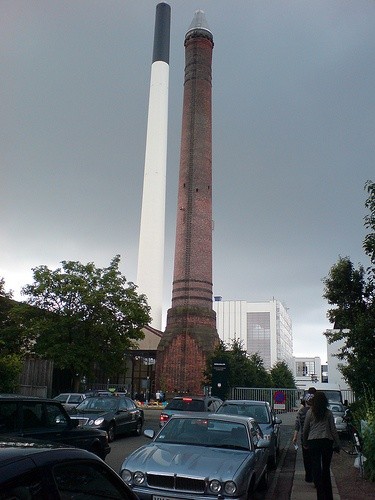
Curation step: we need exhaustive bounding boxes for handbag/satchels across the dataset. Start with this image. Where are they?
[333,441,339,452]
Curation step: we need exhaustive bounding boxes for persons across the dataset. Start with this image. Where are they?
[116,385,128,393]
[292,386,341,500]
[343,400,350,410]
[108,400,116,409]
[250,428,262,446]
[131,385,164,407]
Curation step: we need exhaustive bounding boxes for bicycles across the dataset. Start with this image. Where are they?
[335,408,366,479]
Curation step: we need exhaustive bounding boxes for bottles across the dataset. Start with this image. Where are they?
[293,442,299,450]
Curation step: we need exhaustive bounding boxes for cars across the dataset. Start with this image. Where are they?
[119,410,272,500]
[52,393,101,414]
[0,435,142,500]
[87,388,130,399]
[207,399,282,469]
[159,394,222,430]
[67,395,144,443]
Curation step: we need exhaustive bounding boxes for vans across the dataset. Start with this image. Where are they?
[300,383,349,436]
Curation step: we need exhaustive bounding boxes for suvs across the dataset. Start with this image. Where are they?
[0,393,112,462]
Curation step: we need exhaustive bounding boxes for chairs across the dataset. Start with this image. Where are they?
[255,408,262,415]
[256,412,267,422]
[188,424,208,434]
[230,408,238,414]
[232,427,247,441]
[6,409,40,430]
[94,400,105,407]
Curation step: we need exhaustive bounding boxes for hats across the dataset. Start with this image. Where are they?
[304,393,314,401]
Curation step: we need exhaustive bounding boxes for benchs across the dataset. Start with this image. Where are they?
[197,431,232,443]
